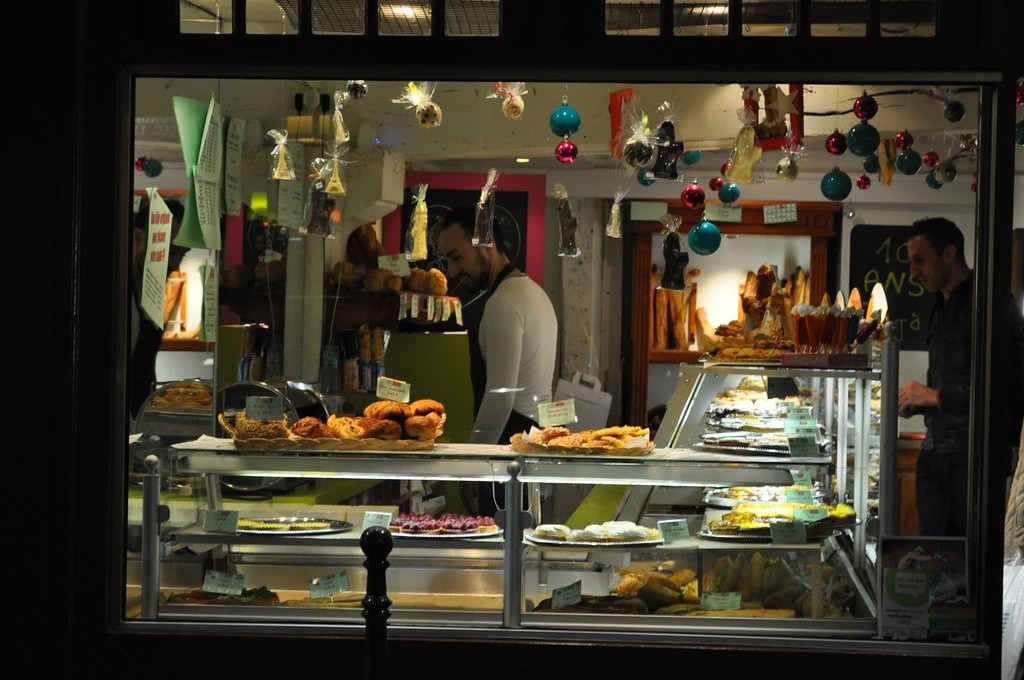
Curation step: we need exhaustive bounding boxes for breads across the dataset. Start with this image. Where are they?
[655,264,888,361]
[615,552,855,622]
[700,377,815,452]
[218,399,444,444]
[181,272,187,329]
[510,426,647,448]
[168,585,369,612]
[331,224,448,295]
[164,271,181,325]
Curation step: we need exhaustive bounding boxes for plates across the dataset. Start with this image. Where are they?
[391,528,505,537]
[690,443,862,540]
[525,533,663,545]
[237,516,355,535]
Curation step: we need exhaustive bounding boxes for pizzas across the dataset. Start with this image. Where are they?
[710,484,856,539]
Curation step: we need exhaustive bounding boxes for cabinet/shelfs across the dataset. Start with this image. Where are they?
[163,339,898,641]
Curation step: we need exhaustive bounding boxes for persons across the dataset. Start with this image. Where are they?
[438,206,559,518]
[130,193,185,424]
[897,217,1024,644]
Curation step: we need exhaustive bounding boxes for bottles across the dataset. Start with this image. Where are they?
[266,336,282,378]
[323,338,342,394]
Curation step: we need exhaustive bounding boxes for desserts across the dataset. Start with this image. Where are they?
[376,512,501,535]
[533,520,659,543]
